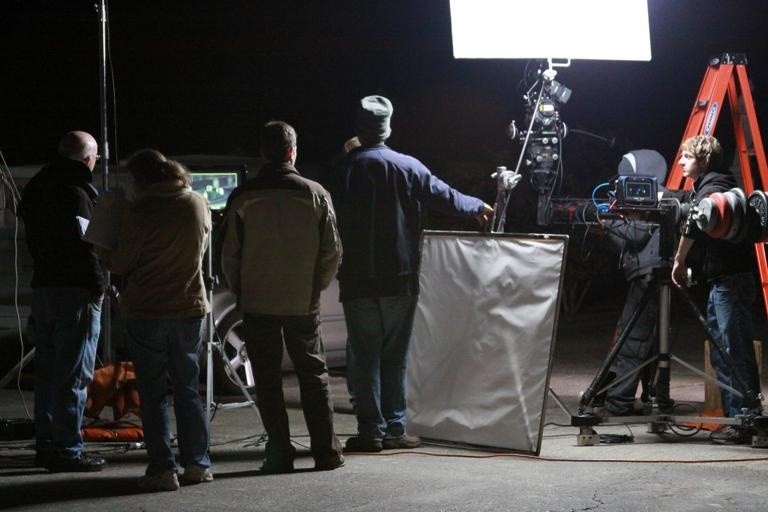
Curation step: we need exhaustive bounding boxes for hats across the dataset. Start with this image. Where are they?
[350,95,393,143]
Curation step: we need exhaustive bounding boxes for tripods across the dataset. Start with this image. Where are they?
[572,227,768,447]
[204,211,263,455]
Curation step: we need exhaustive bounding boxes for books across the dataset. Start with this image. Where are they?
[75,189,135,251]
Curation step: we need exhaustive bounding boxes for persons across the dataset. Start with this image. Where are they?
[12,128,114,473]
[659,134,765,446]
[330,93,497,455]
[594,184,693,418]
[109,147,215,493]
[210,119,346,475]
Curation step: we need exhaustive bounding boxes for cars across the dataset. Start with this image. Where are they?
[200,273,349,395]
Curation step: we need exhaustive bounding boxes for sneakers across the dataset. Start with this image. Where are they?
[316,455,345,470]
[709,425,757,445]
[183,460,213,483]
[133,465,181,490]
[345,434,421,452]
[35,452,106,472]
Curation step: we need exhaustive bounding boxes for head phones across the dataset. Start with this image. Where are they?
[696,136,707,160]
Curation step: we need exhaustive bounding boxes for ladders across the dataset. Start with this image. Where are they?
[612,49,768,348]
